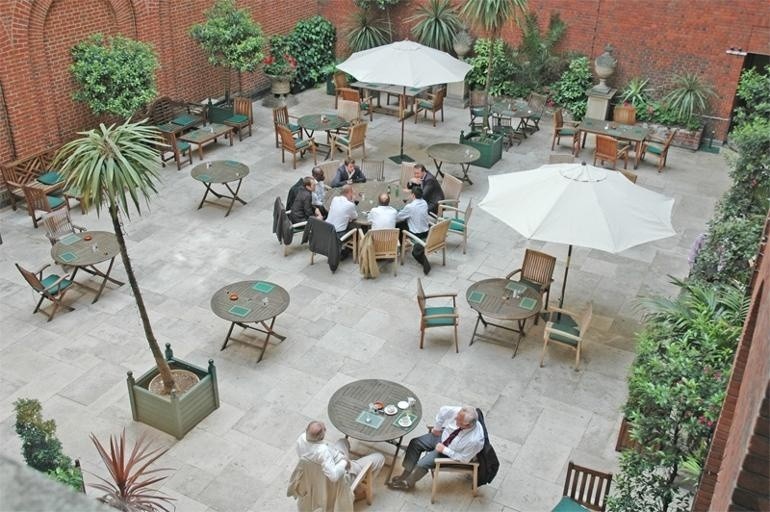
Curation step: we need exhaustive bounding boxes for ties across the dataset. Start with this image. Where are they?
[442,426,464,447]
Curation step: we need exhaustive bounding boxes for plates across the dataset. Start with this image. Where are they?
[384,406,397,415]
[398,419,412,427]
[398,401,409,409]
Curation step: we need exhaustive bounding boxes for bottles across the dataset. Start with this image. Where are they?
[368,402,379,414]
[387,184,391,194]
[512,289,521,300]
[395,185,400,198]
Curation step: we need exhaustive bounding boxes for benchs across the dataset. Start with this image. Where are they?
[0,137,75,211]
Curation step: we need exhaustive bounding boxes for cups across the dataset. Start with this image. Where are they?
[408,397,417,406]
[354,199,359,208]
[365,413,372,422]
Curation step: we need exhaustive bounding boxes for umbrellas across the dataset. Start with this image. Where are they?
[479,161,676,317]
[335,39,474,157]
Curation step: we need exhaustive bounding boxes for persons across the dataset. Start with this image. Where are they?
[298,420,384,502]
[285,177,327,230]
[407,164,445,214]
[388,404,485,491]
[325,185,359,261]
[311,168,326,206]
[368,193,398,229]
[330,158,366,188]
[398,186,429,245]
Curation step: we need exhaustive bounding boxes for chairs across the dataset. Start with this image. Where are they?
[301,456,374,512]
[149,88,253,171]
[548,462,612,512]
[506,248,557,325]
[417,277,458,354]
[21,184,71,228]
[426,425,480,504]
[43,206,88,273]
[470,89,678,172]
[540,303,593,371]
[15,262,76,321]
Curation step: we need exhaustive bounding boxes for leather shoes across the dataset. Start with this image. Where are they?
[392,475,411,490]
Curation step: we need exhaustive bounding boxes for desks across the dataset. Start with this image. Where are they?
[466,278,542,359]
[210,280,291,364]
[52,230,125,305]
[190,160,249,217]
